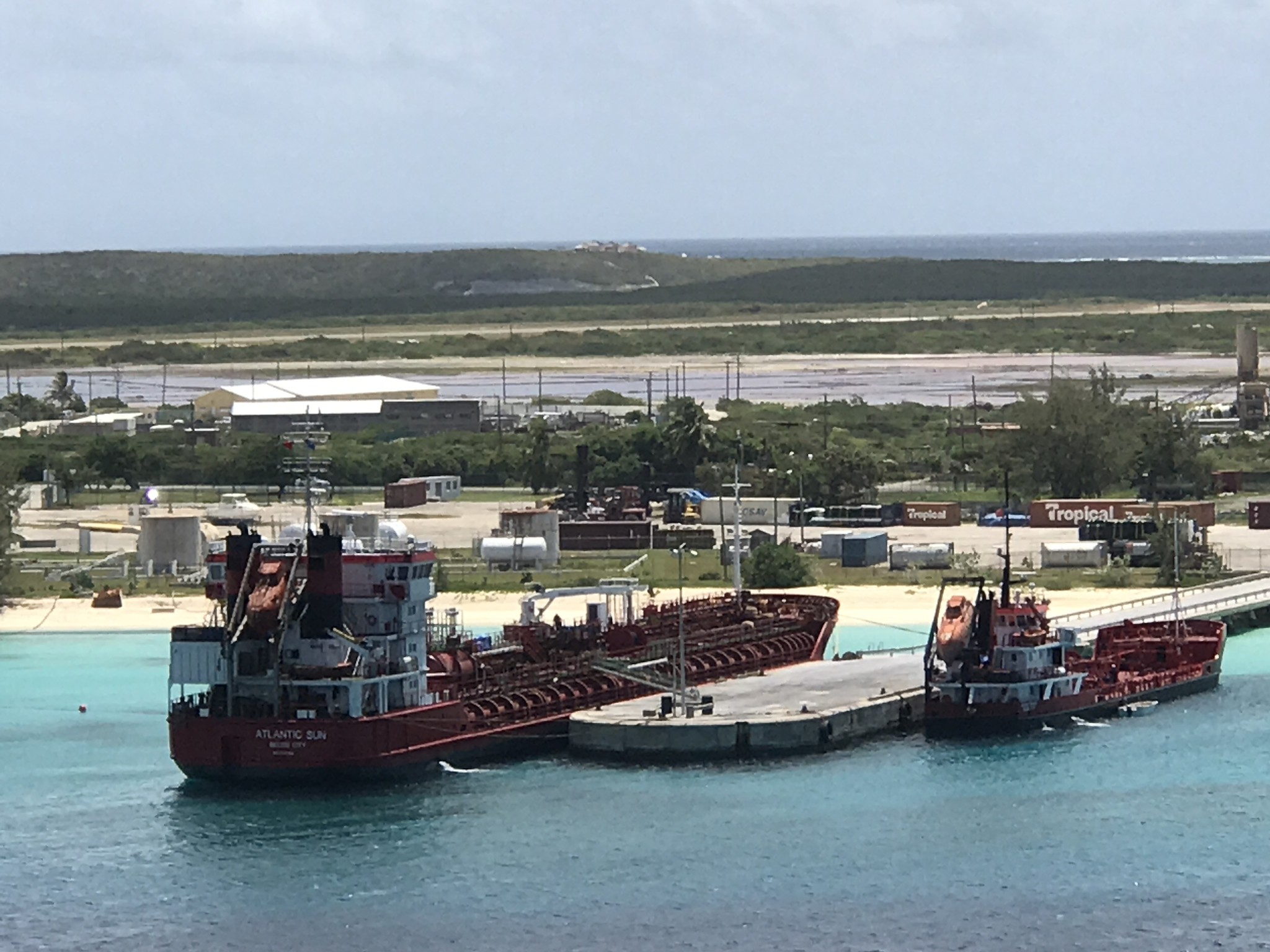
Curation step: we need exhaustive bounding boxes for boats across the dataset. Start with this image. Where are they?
[167,459,841,781]
[920,468,1230,739]
